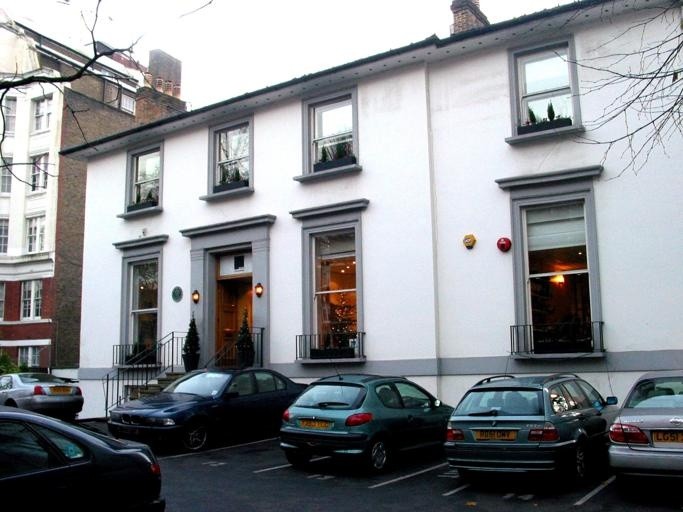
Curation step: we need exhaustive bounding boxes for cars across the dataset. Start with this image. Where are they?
[105,367,310,451]
[607,370,683,481]
[0,404,168,511]
[442,370,622,483]
[0,371,83,420]
[280,373,454,475]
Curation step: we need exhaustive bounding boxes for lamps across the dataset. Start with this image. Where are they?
[189,288,202,305]
[252,280,264,300]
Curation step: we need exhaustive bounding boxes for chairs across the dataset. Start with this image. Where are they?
[377,385,394,407]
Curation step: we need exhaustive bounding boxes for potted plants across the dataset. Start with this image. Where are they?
[180,310,202,374]
[232,310,256,369]
